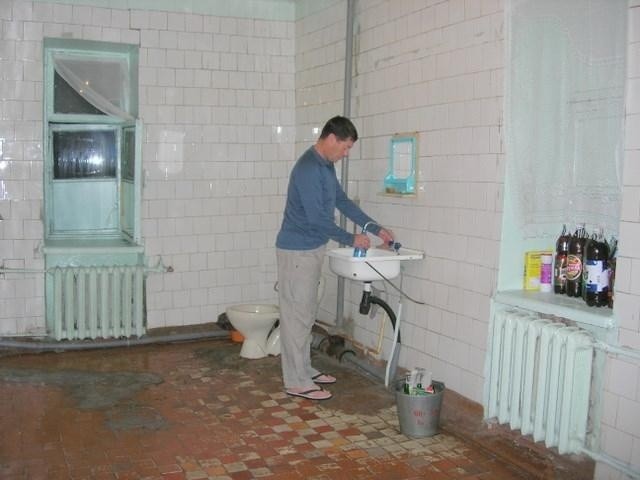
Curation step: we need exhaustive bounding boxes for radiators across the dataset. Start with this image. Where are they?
[482,308,640,479]
[0,263,174,341]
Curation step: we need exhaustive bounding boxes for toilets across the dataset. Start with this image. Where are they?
[227,277,326,359]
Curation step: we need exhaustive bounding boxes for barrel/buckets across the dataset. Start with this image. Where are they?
[552,223,568,294]
[584,226,610,305]
[566,225,585,295]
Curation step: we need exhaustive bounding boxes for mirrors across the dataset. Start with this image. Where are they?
[383,136,417,194]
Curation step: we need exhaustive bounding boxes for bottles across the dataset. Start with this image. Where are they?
[554,221,617,308]
[353,229,366,257]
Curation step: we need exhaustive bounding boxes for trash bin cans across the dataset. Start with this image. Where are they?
[388,377,445,436]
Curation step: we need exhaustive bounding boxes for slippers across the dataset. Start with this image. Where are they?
[310,372,337,384]
[285,384,333,401]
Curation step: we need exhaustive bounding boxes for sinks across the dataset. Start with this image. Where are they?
[325,245,425,281]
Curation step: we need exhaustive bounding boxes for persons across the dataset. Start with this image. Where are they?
[275,116,394,400]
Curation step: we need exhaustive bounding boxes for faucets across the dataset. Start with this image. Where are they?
[361,220,395,247]
